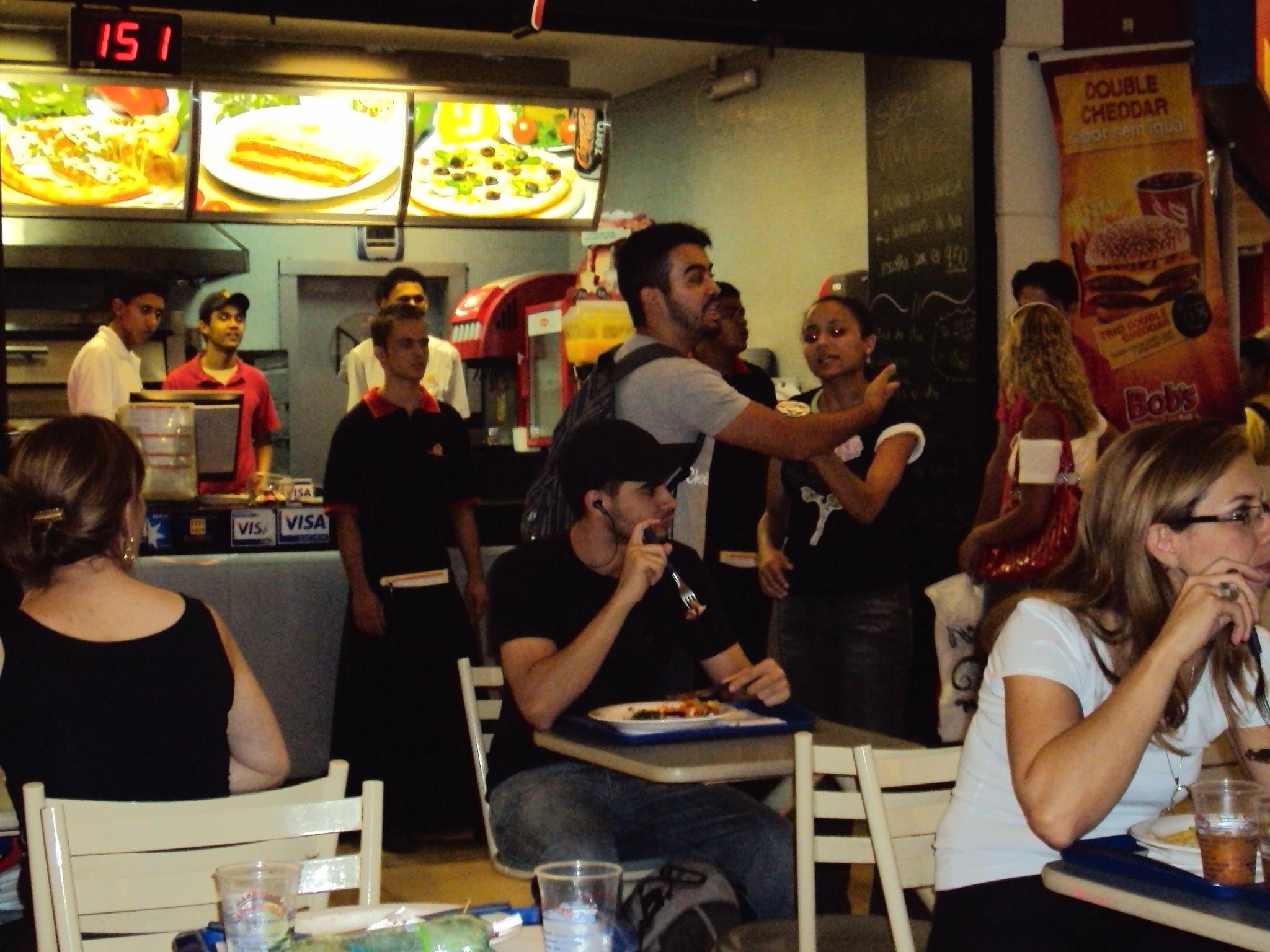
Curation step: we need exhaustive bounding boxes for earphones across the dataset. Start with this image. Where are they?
[593,500,608,514]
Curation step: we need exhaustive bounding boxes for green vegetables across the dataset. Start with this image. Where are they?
[511,105,571,146]
[214,92,301,125]
[0,82,92,129]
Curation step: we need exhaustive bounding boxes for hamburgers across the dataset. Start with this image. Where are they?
[1084,214,1200,323]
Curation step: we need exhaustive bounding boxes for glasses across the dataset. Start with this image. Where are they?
[1160,502,1270,535]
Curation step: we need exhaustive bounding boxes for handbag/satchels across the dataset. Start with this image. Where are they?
[979,407,1084,580]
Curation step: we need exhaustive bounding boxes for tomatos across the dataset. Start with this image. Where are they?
[97,86,168,119]
[513,118,536,145]
[559,119,578,145]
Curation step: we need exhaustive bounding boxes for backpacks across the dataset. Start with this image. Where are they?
[523,341,682,541]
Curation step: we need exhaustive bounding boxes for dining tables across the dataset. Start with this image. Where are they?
[1042,846,1270,952]
[531,717,928,814]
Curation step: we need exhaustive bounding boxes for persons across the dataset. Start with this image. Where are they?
[920,332,1269,952]
[0,411,291,952]
[484,414,806,952]
[66,223,1120,858]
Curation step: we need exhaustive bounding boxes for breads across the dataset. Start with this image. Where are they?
[227,131,367,188]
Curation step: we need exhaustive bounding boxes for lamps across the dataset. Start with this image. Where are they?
[708,66,760,99]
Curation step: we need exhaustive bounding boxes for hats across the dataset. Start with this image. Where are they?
[200,290,251,318]
[556,417,695,490]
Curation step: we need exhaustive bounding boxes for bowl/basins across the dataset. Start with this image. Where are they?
[245,472,294,509]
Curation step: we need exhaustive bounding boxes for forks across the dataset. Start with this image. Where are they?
[1248,626,1270,726]
[643,528,698,610]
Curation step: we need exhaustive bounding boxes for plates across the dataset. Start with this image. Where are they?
[205,495,248,504]
[409,146,585,219]
[587,701,738,735]
[202,105,403,200]
[1127,814,1261,856]
[294,902,521,947]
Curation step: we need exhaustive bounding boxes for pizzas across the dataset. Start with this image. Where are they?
[408,136,571,220]
[0,109,182,202]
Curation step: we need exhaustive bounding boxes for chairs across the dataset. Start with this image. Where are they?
[23,758,350,952]
[456,655,664,903]
[795,731,963,952]
[39,778,385,952]
[852,744,962,951]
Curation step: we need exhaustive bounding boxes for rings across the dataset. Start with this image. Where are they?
[1219,582,1238,602]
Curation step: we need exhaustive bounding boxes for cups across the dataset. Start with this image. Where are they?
[1258,793,1270,886]
[1135,168,1204,287]
[513,427,528,451]
[1190,780,1263,884]
[534,860,623,952]
[775,401,810,417]
[213,861,303,952]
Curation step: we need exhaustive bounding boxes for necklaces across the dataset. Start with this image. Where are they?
[1162,662,1192,808]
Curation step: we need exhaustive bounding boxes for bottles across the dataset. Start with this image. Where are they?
[285,914,497,952]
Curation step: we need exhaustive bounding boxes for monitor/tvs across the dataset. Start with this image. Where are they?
[128,392,243,481]
